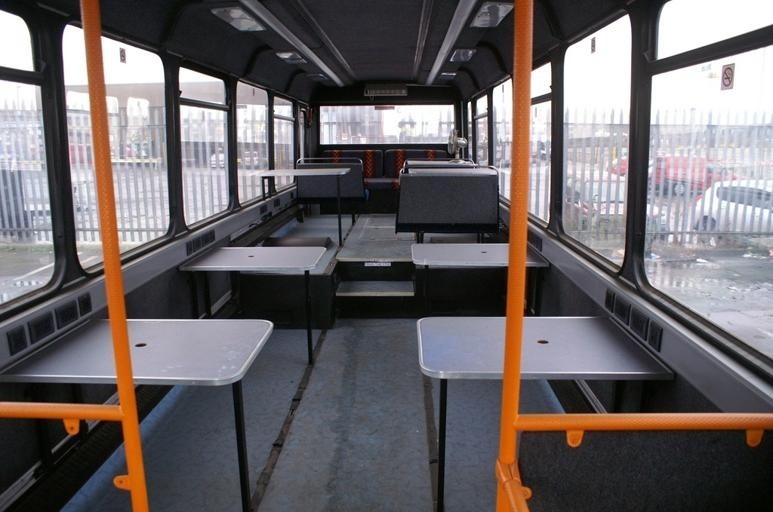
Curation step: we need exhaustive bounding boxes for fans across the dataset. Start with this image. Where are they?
[446,128,467,165]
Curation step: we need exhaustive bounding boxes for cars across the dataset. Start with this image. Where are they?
[67,141,293,170]
[565,152,773,245]
[463,132,555,171]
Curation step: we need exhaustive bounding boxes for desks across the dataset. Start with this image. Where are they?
[394,159,499,245]
[257,167,352,248]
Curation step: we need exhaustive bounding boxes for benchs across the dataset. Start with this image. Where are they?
[295,143,468,226]
